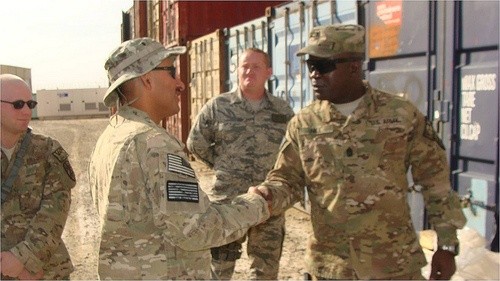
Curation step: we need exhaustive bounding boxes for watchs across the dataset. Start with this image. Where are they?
[438,244,460,255]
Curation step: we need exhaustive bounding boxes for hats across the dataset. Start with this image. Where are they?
[103,37,186,107]
[296,24,365,58]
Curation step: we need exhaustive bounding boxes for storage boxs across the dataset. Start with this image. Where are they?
[120,0,500,251]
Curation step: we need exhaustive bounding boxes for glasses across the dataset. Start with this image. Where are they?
[153,67,176,79]
[306,57,365,73]
[0,100,38,110]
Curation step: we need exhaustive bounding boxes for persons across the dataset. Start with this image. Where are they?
[88,36,274,281]
[0,74,76,281]
[186,48,295,281]
[257,24,468,281]
